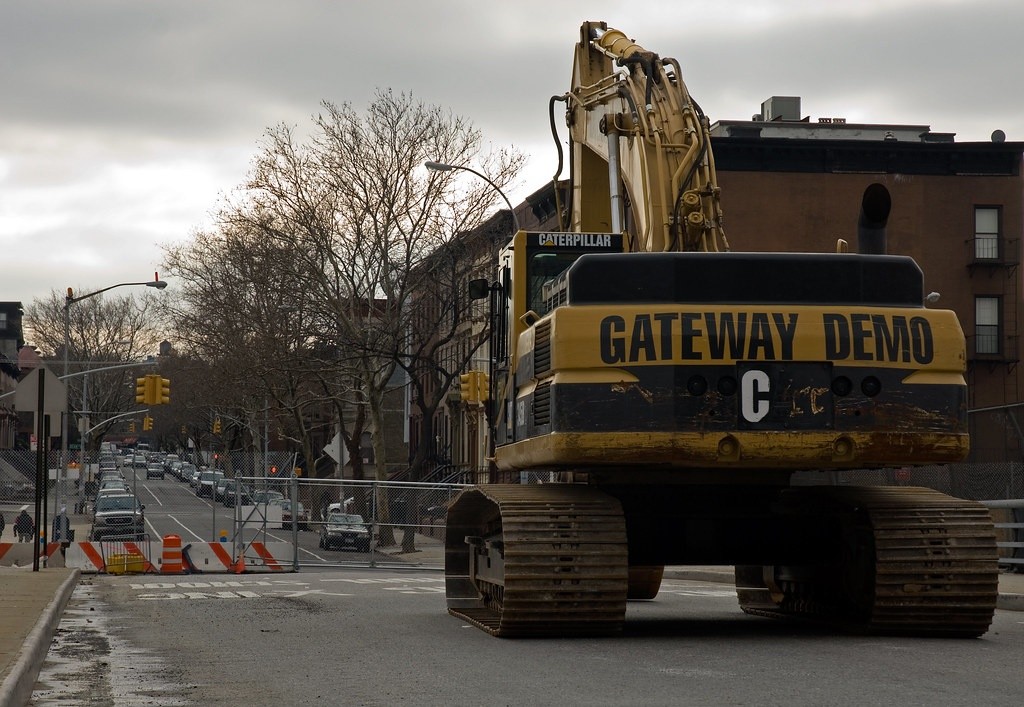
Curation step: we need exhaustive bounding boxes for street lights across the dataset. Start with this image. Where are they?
[426,161,521,232]
[78,341,130,514]
[59,281,168,540]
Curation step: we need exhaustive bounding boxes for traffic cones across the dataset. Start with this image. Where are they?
[233,549,247,574]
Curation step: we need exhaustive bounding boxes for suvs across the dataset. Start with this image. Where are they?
[92,492,146,541]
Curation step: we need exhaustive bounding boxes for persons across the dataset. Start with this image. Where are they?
[320,487,330,519]
[52,508,70,541]
[0,514,5,536]
[367,494,377,519]
[13,510,34,543]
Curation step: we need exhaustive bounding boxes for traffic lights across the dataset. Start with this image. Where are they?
[213,420,221,434]
[460,371,478,400]
[135,375,153,405]
[143,417,153,431]
[214,454,219,459]
[129,423,134,433]
[270,466,277,473]
[153,375,170,405]
[477,372,493,401]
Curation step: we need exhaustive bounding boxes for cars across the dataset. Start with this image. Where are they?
[189,472,200,488]
[249,490,283,506]
[268,499,283,506]
[196,471,226,498]
[319,513,371,553]
[271,501,310,532]
[97,449,130,498]
[131,456,147,469]
[321,493,369,521]
[222,483,250,508]
[145,451,196,483]
[210,479,235,501]
[123,455,133,467]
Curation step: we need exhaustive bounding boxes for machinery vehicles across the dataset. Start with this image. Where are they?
[446,21,999,640]
